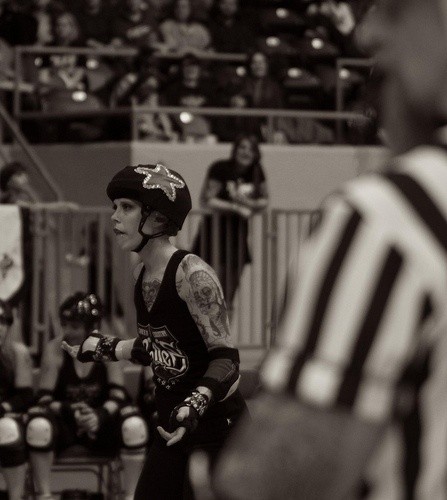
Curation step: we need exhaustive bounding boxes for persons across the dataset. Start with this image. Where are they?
[60,162,250,500]
[65,220,126,338]
[0,0,388,146]
[26,289,147,500]
[191,131,269,321]
[0,163,54,346]
[0,300,32,500]
[220,0,447,500]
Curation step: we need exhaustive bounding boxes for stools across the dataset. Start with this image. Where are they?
[24,432,120,500]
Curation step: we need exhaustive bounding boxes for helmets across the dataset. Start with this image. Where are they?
[0,299,14,325]
[105,164,192,231]
[59,291,102,327]
